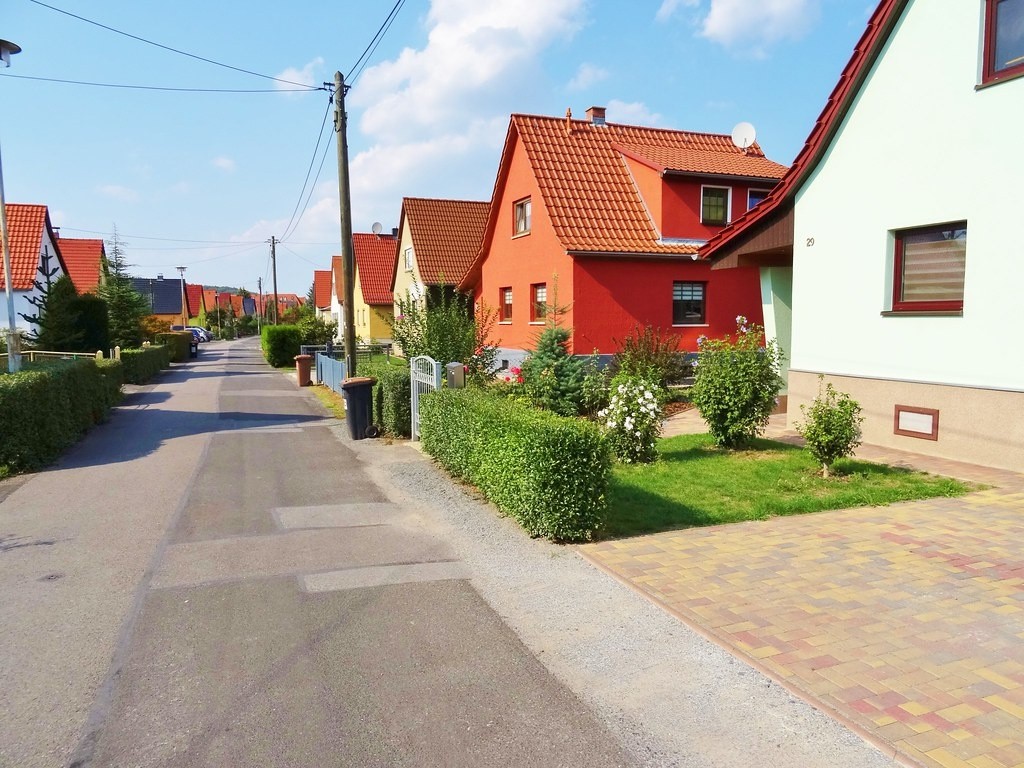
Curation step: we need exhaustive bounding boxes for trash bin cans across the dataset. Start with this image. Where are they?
[338,375,379,441]
[190,341,198,358]
[294,354,313,387]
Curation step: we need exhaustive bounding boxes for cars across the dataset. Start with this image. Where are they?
[172,325,216,345]
